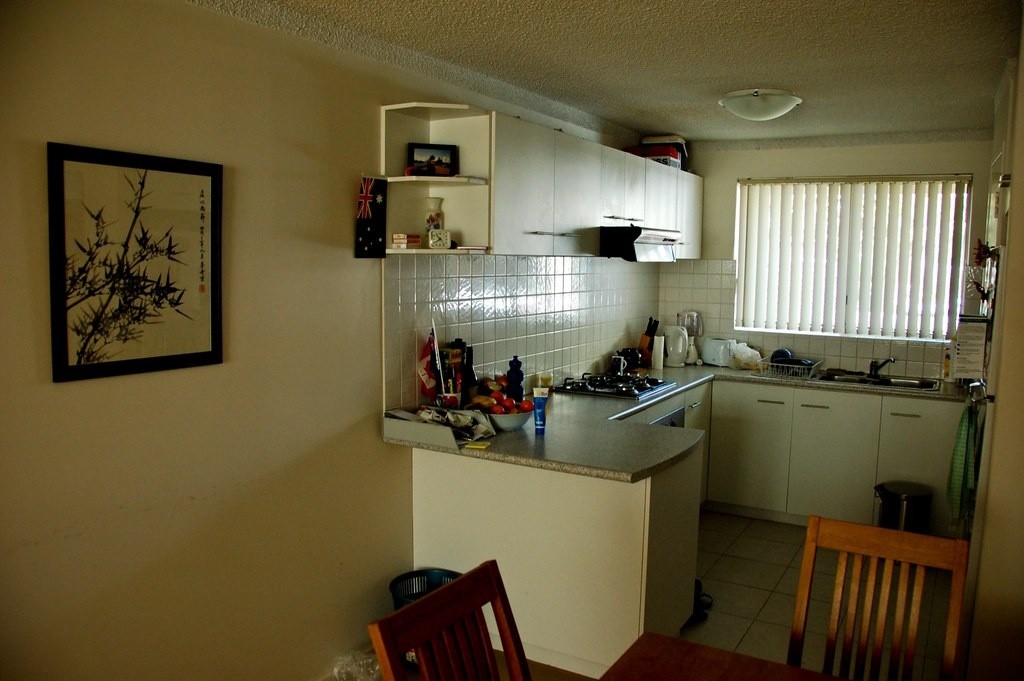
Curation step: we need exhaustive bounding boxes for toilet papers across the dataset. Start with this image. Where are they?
[652,336,664,369]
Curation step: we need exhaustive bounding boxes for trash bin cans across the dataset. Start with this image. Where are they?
[875,481,932,568]
[390,567,463,675]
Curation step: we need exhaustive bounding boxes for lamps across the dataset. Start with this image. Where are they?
[718,89,802,120]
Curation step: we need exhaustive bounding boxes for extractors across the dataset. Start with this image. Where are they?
[600,227,682,263]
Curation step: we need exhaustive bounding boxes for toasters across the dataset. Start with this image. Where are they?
[702,337,736,366]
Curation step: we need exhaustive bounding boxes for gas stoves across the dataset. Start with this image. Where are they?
[555,372,677,399]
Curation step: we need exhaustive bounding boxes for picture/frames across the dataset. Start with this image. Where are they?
[46,141,224,383]
[407,142,459,177]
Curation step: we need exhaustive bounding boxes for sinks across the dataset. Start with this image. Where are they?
[871,375,940,391]
[816,374,876,385]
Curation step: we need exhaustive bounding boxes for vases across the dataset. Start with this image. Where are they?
[425,197,443,248]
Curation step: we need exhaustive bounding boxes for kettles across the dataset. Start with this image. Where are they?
[662,326,688,368]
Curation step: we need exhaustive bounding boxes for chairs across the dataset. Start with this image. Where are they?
[787,515,969,681]
[367,560,532,681]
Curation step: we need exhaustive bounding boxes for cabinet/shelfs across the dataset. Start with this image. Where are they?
[379,102,702,259]
[412,381,973,681]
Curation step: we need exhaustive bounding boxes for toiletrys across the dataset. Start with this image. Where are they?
[534,397,548,435]
[533,387,549,416]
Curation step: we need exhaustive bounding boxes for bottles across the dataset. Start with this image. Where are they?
[450,339,466,394]
[424,197,444,248]
[466,347,478,404]
[506,356,524,408]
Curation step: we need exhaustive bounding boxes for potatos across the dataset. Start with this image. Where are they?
[472,395,497,407]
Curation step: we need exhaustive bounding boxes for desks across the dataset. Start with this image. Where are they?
[598,631,844,681]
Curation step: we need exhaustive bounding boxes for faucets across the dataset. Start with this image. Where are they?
[869,357,896,378]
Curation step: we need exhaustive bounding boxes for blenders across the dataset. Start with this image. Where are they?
[677,311,702,364]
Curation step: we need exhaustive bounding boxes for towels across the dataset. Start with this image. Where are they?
[945,395,980,522]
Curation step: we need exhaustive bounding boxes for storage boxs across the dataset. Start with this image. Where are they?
[642,135,688,171]
[625,145,681,170]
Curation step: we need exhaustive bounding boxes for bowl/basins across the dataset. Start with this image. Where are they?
[771,348,792,367]
[488,410,534,431]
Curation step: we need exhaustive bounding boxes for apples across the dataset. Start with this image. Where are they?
[497,374,508,387]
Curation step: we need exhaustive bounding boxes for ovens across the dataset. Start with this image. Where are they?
[649,406,685,428]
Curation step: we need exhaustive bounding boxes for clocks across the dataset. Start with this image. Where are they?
[429,229,451,249]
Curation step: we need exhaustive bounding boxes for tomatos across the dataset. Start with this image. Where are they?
[489,391,533,415]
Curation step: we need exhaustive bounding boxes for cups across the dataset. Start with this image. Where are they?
[610,356,627,376]
[436,392,461,410]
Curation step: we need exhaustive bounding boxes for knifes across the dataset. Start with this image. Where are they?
[645,316,659,352]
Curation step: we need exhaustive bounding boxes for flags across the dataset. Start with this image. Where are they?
[352,177,387,259]
[417,327,440,398]
